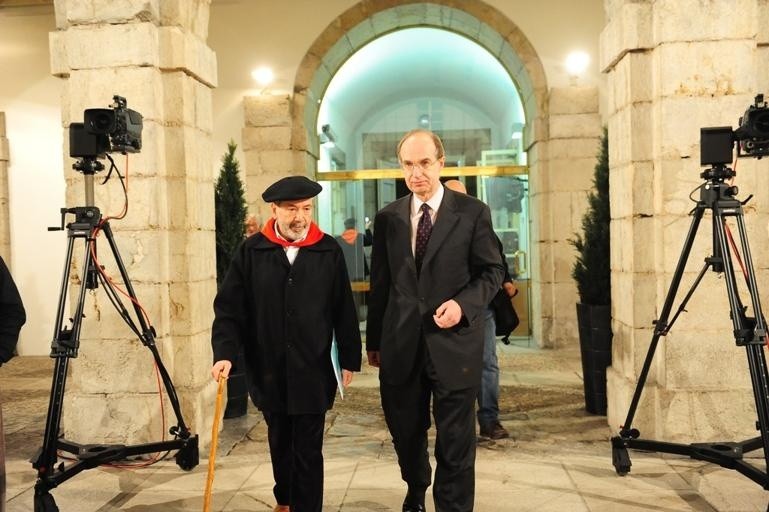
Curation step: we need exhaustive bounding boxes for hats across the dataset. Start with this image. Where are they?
[261,175,324,203]
[344,218,356,225]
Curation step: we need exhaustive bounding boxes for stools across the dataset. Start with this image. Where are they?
[348,282,372,324]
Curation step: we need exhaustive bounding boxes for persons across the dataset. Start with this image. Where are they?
[443,178,518,440]
[0,256,26,512]
[367,131,508,512]
[211,178,362,511]
[334,217,374,290]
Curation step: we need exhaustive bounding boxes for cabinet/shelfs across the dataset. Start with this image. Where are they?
[508,277,532,337]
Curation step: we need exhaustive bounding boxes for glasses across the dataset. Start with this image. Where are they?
[402,154,443,170]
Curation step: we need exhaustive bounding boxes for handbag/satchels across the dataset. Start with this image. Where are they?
[487,288,520,345]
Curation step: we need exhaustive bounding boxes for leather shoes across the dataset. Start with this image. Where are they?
[401,483,428,512]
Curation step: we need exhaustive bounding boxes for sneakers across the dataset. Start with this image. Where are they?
[479,421,510,440]
[272,504,290,512]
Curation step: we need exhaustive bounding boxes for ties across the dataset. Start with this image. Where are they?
[414,202,433,280]
